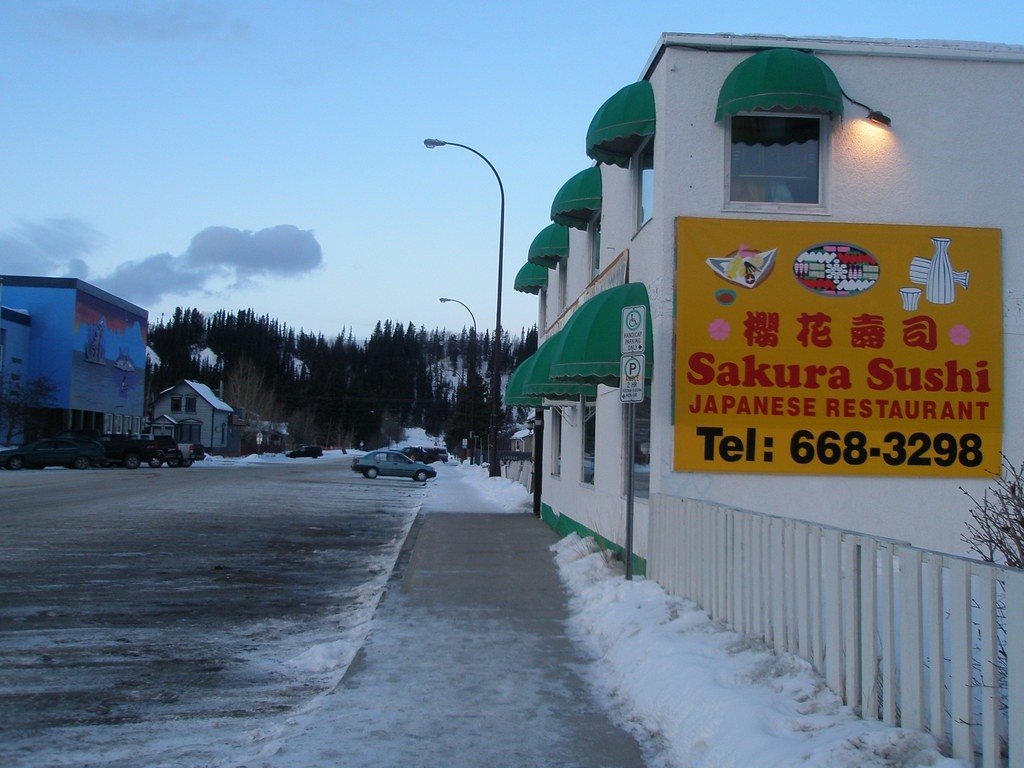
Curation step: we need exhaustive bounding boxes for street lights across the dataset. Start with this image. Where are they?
[424,138,505,478]
[439,297,477,466]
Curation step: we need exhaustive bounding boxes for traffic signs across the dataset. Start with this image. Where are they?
[621,305,645,355]
[620,354,645,403]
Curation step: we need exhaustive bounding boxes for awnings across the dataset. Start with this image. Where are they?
[514,262,548,295]
[586,81,656,169]
[714,47,844,123]
[551,166,602,232]
[528,223,569,270]
[504,355,550,410]
[521,330,597,401]
[549,282,653,389]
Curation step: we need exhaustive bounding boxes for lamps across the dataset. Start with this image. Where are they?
[527,417,536,432]
[534,414,542,428]
[869,115,891,127]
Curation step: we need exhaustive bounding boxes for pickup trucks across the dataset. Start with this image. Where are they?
[58,428,206,469]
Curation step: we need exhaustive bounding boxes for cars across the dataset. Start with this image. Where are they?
[388,444,448,465]
[350,450,436,482]
[0,437,108,470]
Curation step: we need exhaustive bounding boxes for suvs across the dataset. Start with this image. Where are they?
[284,446,324,459]
